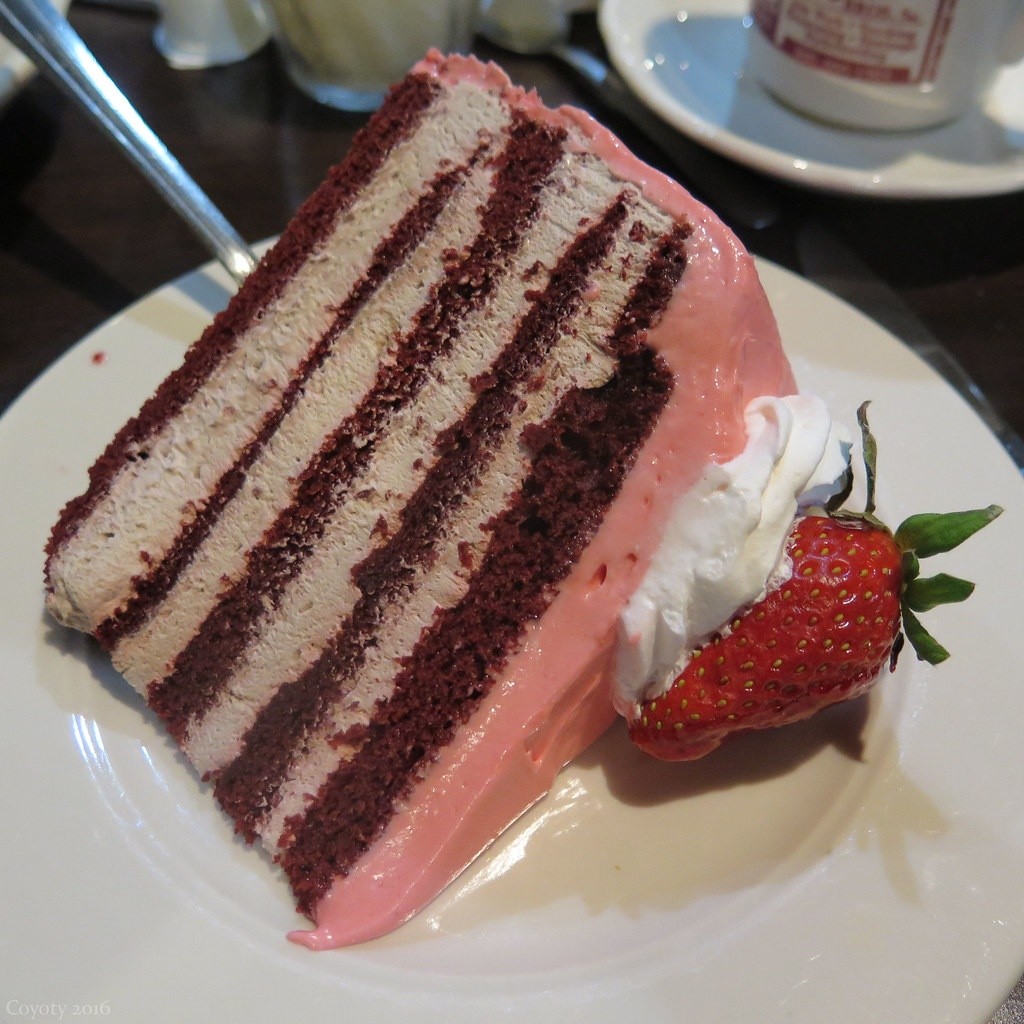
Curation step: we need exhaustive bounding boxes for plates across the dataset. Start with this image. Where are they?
[1,232,1024,1022]
[596,0,1024,199]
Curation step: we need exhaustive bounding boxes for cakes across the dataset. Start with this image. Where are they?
[37,44,1001,954]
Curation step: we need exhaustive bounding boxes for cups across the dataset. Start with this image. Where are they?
[744,0,1001,132]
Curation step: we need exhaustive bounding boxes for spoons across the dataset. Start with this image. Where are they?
[474,0,778,228]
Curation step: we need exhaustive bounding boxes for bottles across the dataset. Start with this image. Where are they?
[152,0,270,69]
[261,0,474,112]
